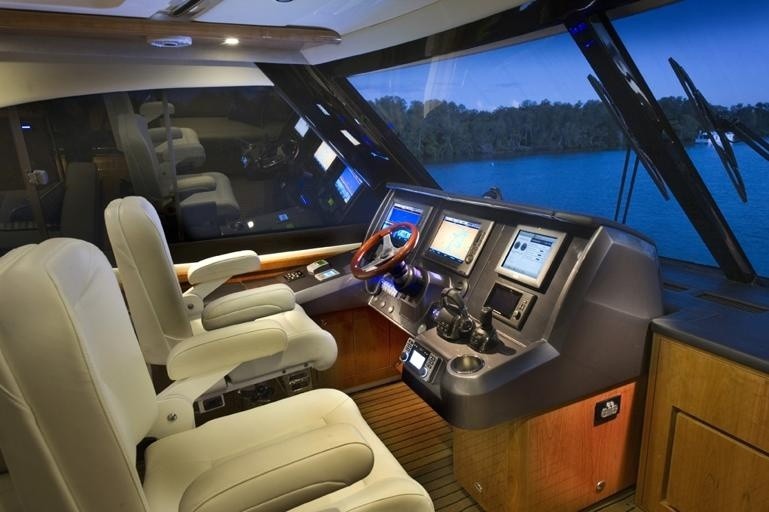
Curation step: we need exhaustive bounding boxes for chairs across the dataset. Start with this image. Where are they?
[0,192,436,511]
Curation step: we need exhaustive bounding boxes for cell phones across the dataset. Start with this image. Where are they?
[306,259,329,273]
[314,268,340,281]
